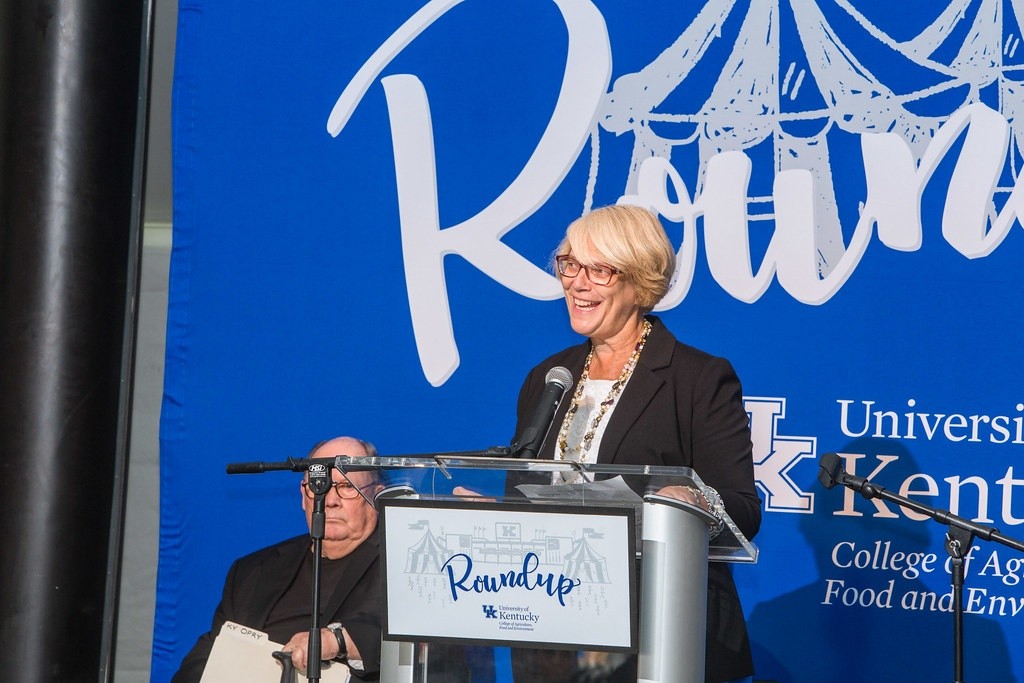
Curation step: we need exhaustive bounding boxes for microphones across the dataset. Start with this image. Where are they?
[518,366,573,460]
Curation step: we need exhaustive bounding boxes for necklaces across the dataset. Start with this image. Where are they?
[559,318,653,483]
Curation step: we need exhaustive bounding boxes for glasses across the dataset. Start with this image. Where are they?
[557,254,625,286]
[302,482,374,499]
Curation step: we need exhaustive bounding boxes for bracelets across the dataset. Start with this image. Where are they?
[684,486,701,505]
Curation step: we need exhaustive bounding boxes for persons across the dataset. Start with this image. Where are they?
[170,436,471,683]
[452,204,762,683]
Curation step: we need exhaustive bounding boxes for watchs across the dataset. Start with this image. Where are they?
[326,622,348,661]
